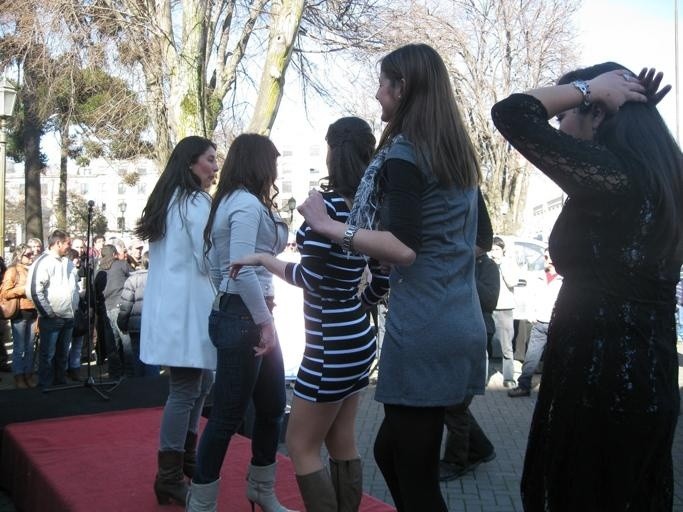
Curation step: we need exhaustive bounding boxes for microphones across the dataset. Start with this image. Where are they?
[87,199,96,218]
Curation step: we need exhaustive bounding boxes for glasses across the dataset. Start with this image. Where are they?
[23,252,34,259]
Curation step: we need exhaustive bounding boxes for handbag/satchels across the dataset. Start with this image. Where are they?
[0,291,20,320]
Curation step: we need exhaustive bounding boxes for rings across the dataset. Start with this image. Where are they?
[622,72,633,83]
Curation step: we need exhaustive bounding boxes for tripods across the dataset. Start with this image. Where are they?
[42,215,121,401]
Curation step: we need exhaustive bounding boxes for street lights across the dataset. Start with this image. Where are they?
[116,197,126,238]
[285,197,296,223]
[0,66,17,259]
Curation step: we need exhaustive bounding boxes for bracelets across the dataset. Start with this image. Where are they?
[342,224,360,254]
[569,79,592,113]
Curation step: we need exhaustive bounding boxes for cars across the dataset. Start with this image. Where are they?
[498,234,567,284]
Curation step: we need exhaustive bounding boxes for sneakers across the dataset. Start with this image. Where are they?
[504,373,539,397]
[1,354,138,391]
[440,446,496,480]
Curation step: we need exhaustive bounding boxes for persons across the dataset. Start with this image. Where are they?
[265,232,683,398]
[491,62,683,512]
[0,231,160,394]
[225,116,391,512]
[181,134,300,512]
[297,43,488,511]
[131,134,219,506]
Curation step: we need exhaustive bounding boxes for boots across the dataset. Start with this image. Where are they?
[154,439,362,512]
